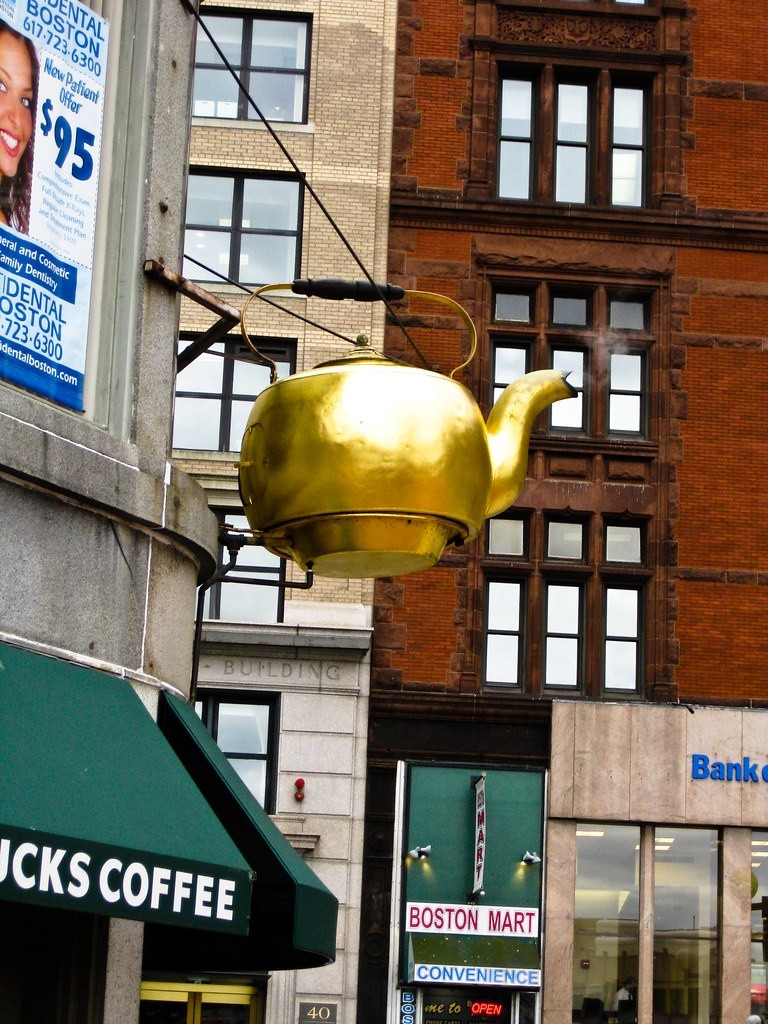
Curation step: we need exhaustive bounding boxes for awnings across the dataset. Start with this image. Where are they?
[0,641,257,937]
[140,690,341,974]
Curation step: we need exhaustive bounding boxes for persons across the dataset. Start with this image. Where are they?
[1,19,40,235]
[609,976,637,1024]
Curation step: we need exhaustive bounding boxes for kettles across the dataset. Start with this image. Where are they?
[235,275,586,582]
